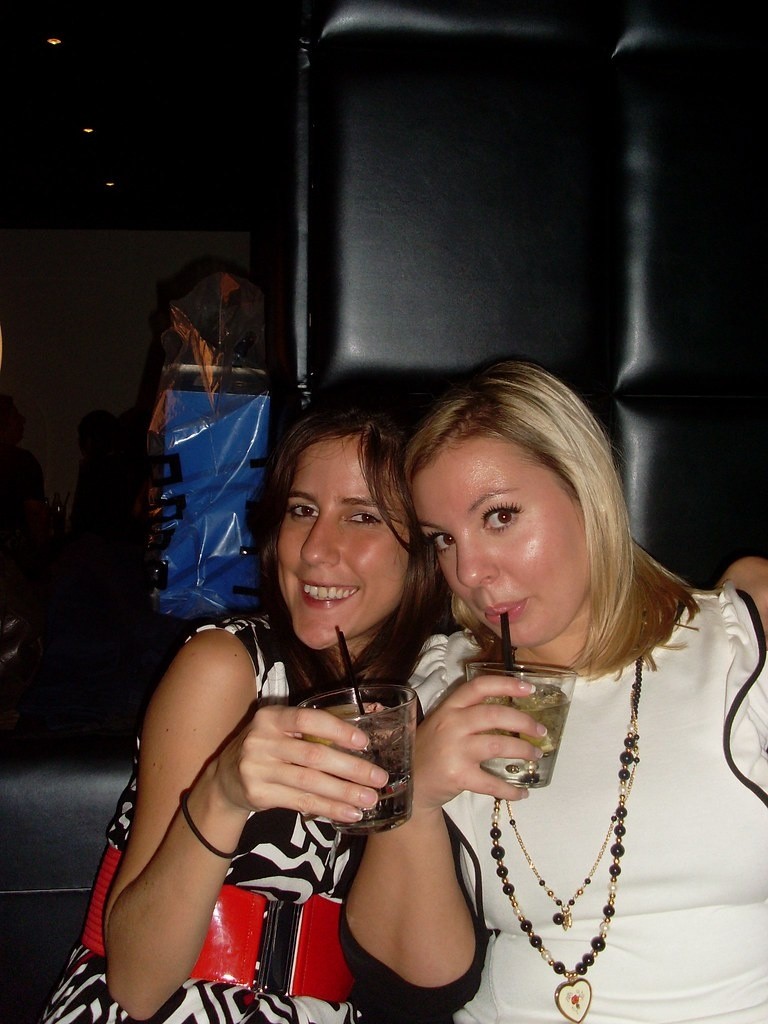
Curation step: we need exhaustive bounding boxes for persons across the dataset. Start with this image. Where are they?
[0,392,50,604]
[335,359,767,1024]
[42,365,768,1024]
[54,408,152,725]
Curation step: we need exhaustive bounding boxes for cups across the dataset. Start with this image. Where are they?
[463,662,578,790]
[296,684,417,835]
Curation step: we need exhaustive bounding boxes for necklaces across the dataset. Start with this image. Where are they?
[473,644,642,1024]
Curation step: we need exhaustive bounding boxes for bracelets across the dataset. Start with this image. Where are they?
[180,789,241,859]
